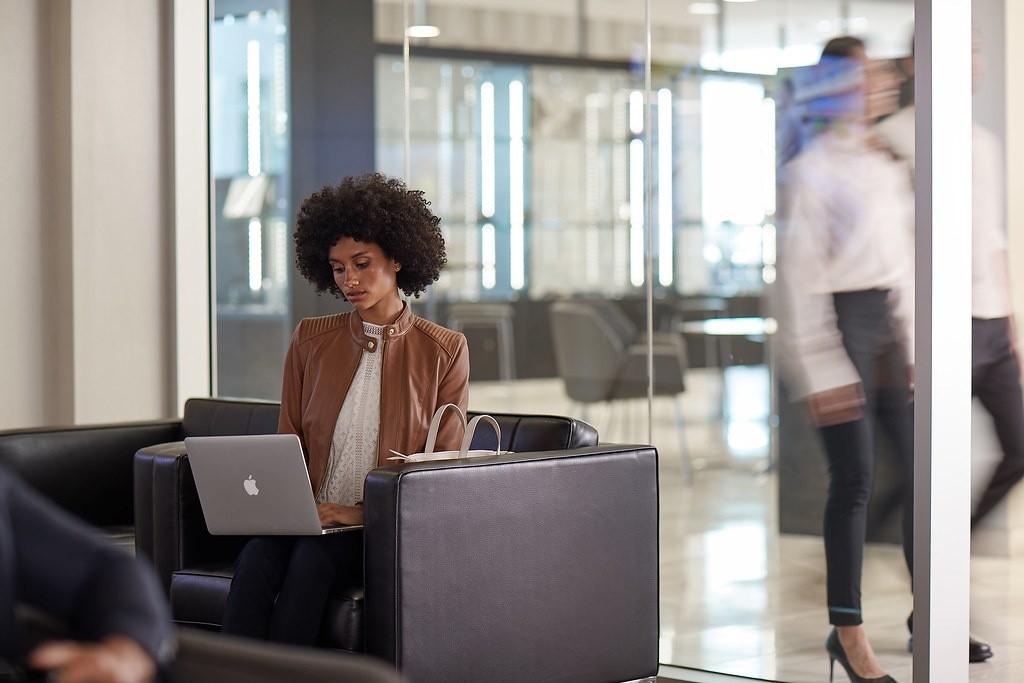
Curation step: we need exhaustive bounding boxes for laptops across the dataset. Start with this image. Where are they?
[183,434,364,535]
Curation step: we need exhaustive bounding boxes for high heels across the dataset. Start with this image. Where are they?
[908,610,993,663]
[825,626,898,683]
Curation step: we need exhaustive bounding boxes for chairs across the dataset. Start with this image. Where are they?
[583,297,690,443]
[552,305,687,478]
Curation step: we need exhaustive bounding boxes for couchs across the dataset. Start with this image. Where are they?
[0,397,281,557]
[134,411,660,683]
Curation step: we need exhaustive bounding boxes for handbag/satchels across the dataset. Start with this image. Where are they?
[387,403,514,464]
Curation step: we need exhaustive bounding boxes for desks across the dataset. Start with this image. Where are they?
[682,317,780,424]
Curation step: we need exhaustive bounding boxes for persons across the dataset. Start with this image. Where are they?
[779,37,1024,683]
[219,174,470,643]
[0,464,179,683]
